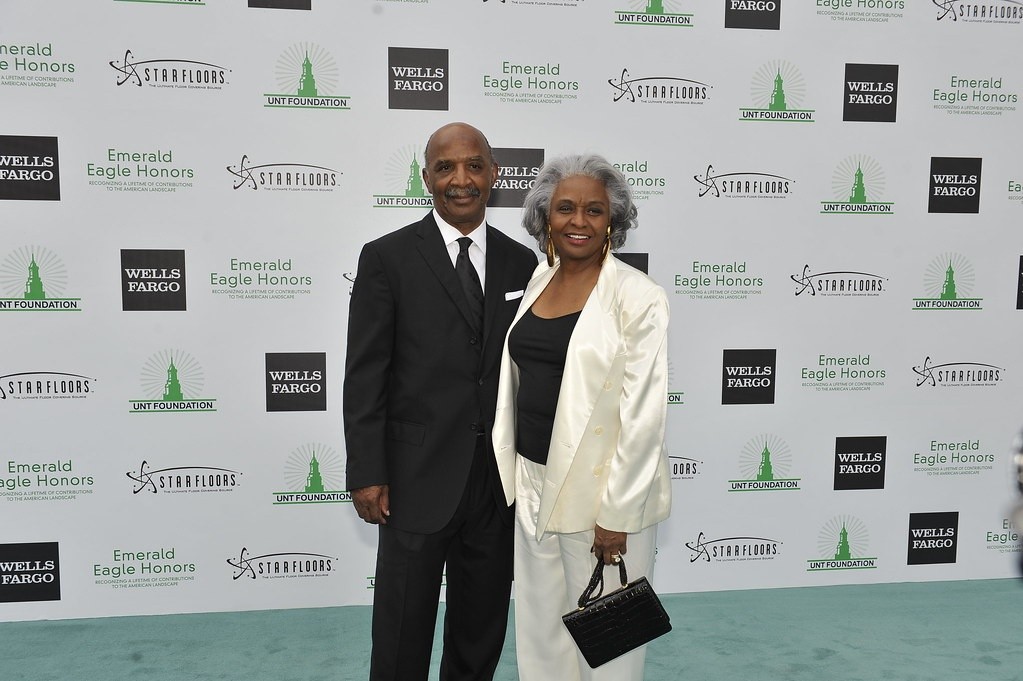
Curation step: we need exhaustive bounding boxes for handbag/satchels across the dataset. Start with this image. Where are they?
[562,552,672,669]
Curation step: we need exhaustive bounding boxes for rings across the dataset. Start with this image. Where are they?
[611,552,620,563]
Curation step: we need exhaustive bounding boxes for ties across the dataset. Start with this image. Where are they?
[455,237,484,345]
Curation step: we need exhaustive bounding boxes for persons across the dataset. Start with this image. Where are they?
[342,118,540,681]
[488,151,673,681]
